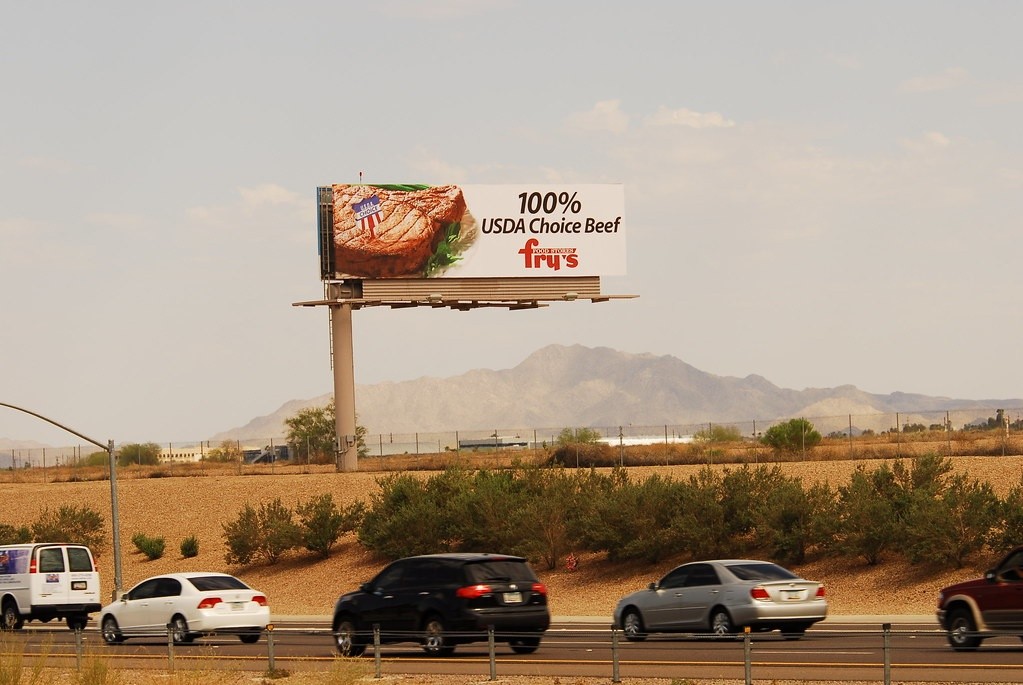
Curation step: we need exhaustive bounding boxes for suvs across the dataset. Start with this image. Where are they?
[329,551,552,658]
[934,546,1023,652]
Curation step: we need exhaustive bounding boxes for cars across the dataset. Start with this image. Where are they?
[609,558,830,640]
[96,570,272,648]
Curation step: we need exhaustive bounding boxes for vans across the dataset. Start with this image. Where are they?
[0,540,102,631]
